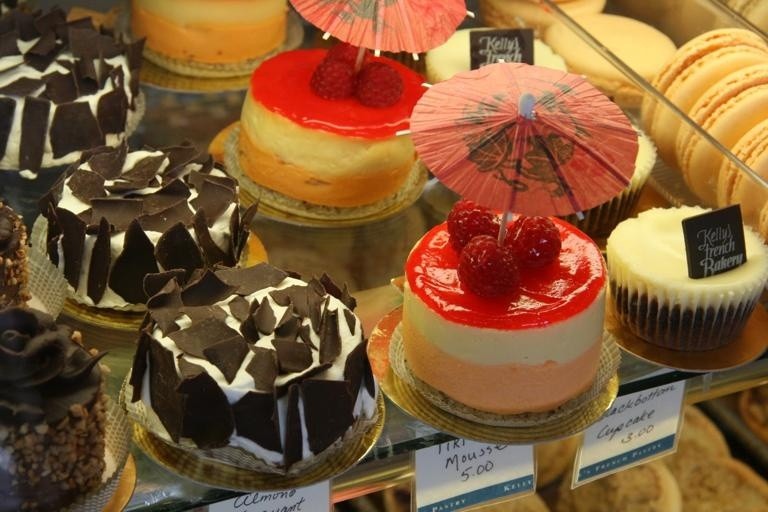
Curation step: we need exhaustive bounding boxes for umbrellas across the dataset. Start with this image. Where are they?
[289,0,474,73]
[396,64,642,245]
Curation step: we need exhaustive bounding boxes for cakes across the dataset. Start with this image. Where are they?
[37,138,259,312]
[0,308,111,512]
[608,207,768,351]
[240,41,428,207]
[425,27,567,84]
[546,13,678,110]
[641,27,768,241]
[0,201,33,309]
[554,123,657,238]
[129,261,380,472]
[0,17,147,190]
[402,199,607,414]
[482,0,606,41]
[130,0,288,64]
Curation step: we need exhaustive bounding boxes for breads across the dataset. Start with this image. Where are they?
[458,387,768,512]
